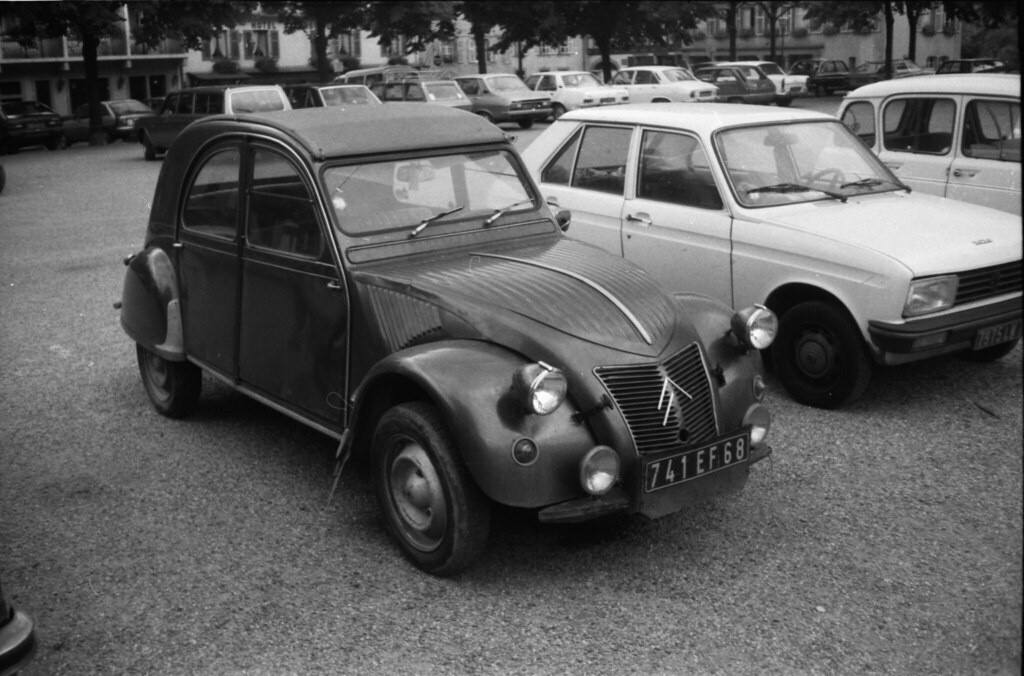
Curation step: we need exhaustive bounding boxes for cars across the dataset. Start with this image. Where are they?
[451,74,554,127]
[879,59,935,76]
[936,58,1011,73]
[606,66,720,104]
[111,103,777,578]
[809,57,1022,214]
[693,64,778,104]
[525,71,629,120]
[368,70,473,113]
[283,82,384,109]
[704,61,810,106]
[60,98,154,145]
[0,95,67,152]
[473,100,1023,411]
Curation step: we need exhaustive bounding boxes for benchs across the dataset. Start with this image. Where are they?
[865,131,954,150]
[186,182,309,239]
[580,166,714,206]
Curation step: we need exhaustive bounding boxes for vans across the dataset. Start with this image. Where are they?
[332,64,424,99]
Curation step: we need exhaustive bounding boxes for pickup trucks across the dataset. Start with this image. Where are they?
[787,58,887,97]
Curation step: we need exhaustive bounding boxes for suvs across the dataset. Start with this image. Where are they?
[134,84,293,161]
